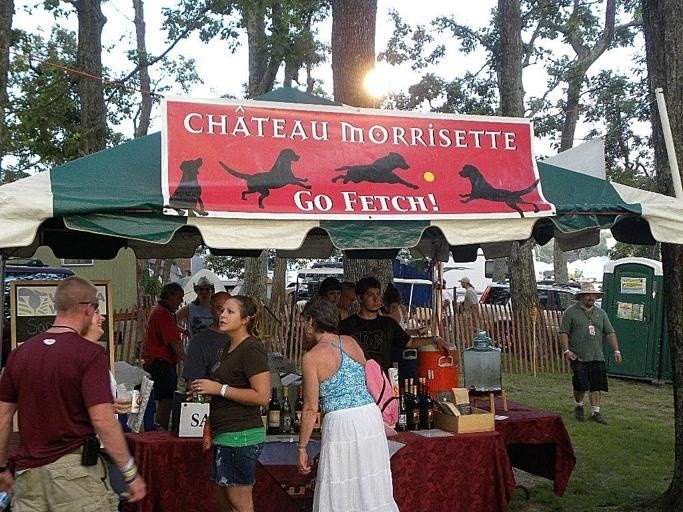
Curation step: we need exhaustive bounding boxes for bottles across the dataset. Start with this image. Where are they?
[0,490,10,512]
[397,377,433,430]
[261,385,322,436]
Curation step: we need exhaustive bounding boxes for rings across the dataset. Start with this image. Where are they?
[301,469,303,471]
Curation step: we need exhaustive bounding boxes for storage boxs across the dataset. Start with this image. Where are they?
[172,403,210,438]
[433,403,494,434]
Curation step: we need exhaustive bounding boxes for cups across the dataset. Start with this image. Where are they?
[191,389,205,403]
[114,383,136,414]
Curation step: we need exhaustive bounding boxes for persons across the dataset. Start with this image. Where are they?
[145,276,231,428]
[298,296,398,512]
[559,282,622,425]
[443,276,478,311]
[190,293,271,511]
[317,276,451,384]
[2,276,146,511]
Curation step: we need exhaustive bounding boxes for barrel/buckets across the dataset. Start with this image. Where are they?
[418,343,460,403]
[401,347,418,388]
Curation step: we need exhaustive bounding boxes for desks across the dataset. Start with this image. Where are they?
[116,430,517,512]
[471,394,575,501]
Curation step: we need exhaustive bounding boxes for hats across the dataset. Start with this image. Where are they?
[194,276,215,291]
[458,276,470,283]
[573,282,604,301]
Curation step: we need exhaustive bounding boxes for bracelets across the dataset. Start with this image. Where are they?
[563,349,569,354]
[614,351,621,354]
[298,446,307,448]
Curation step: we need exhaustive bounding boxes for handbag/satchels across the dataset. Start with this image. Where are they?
[364,357,399,427]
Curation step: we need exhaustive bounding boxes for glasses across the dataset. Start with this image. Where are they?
[78,300,100,311]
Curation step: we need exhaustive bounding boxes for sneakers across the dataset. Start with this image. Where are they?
[575,404,585,422]
[590,411,607,426]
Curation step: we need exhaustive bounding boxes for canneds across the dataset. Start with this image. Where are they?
[192,391,204,404]
[0,490,11,511]
[130,390,140,415]
